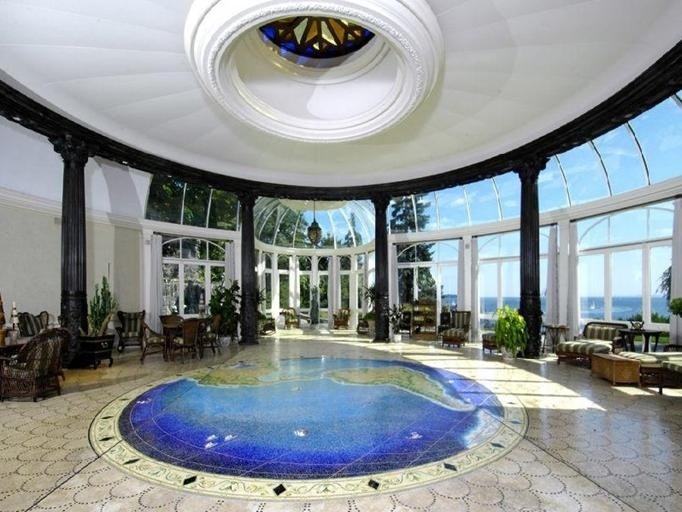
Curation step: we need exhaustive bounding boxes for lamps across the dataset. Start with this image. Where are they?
[306,199,323,245]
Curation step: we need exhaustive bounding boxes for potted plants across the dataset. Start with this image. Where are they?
[76,276,120,369]
[491,304,529,358]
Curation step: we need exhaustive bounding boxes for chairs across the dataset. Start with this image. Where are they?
[115,308,299,364]
[542,321,682,396]
[0,305,75,405]
[332,302,507,357]
